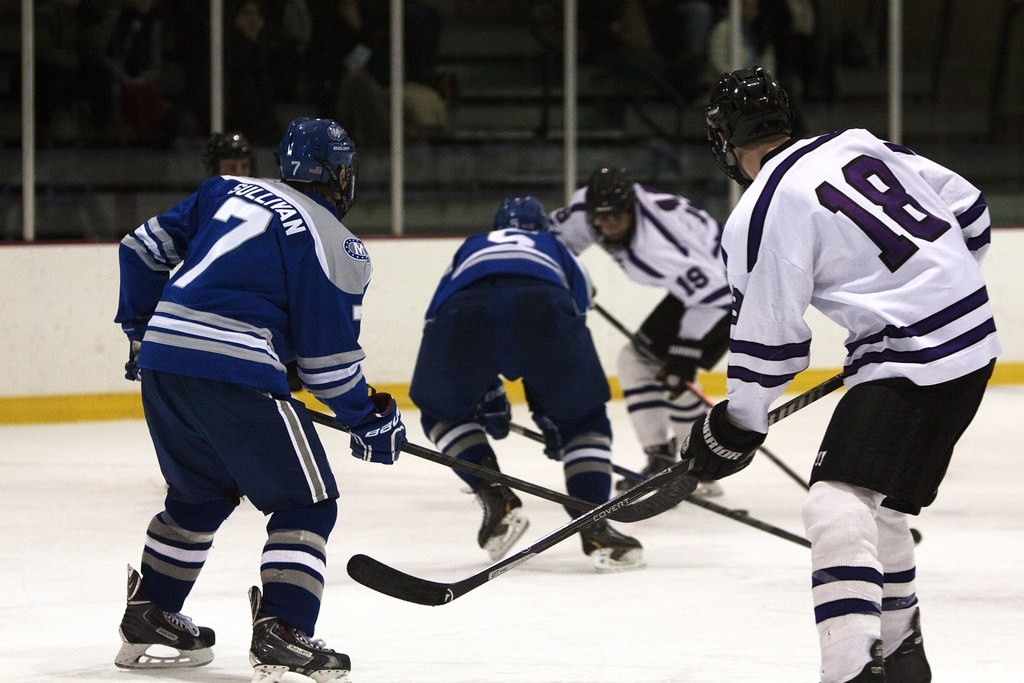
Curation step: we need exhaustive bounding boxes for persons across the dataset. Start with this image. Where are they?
[201,130,256,180]
[0,0,453,149]
[113,117,407,683]
[552,167,731,497]
[515,0,860,105]
[408,196,647,573]
[681,67,1003,683]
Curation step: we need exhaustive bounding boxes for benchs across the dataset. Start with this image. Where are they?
[0,27,1024,243]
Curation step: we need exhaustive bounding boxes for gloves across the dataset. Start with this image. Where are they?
[681,397,766,482]
[125,335,145,381]
[656,354,698,402]
[483,402,512,438]
[352,391,407,465]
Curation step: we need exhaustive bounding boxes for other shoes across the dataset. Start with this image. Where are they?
[841,640,887,683]
[884,606,932,683]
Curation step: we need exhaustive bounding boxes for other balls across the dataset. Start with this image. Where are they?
[734,510,747,515]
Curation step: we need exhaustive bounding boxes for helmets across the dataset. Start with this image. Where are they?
[203,131,258,179]
[275,117,358,217]
[705,66,795,184]
[495,193,549,230]
[586,164,635,252]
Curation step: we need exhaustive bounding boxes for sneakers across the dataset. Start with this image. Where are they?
[247,585,352,683]
[615,438,680,492]
[116,564,216,669]
[567,506,644,571]
[462,456,529,564]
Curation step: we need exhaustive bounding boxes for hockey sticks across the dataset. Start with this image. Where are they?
[344,372,848,607]
[593,303,922,547]
[510,418,811,548]
[299,401,701,524]
